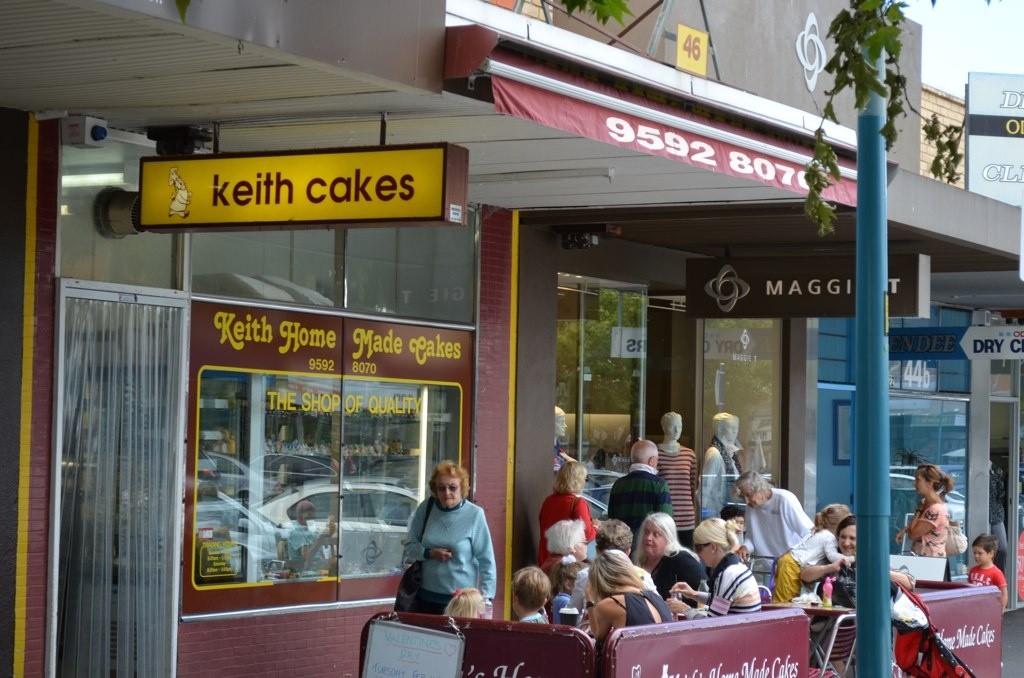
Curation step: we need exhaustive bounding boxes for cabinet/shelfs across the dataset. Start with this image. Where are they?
[248,390,451,461]
[70,392,235,574]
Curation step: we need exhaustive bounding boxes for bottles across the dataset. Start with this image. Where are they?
[822,575,837,608]
[667,590,684,619]
[696,580,712,611]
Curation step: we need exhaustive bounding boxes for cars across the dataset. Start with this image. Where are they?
[883,460,969,544]
[575,469,769,530]
[192,446,436,578]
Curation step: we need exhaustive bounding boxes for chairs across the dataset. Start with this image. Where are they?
[806,614,860,678]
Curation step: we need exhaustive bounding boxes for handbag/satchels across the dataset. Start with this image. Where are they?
[394,494,436,613]
[945,520,968,555]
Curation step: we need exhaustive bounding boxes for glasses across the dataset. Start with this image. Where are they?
[434,482,461,491]
[585,475,588,482]
[695,543,710,552]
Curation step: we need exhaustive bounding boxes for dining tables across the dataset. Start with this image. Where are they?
[761,603,860,674]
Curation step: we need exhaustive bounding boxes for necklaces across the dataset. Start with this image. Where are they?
[439,500,462,532]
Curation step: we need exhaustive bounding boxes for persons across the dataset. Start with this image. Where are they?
[654,411,699,551]
[988,459,1009,580]
[286,499,337,578]
[441,503,917,678]
[735,470,815,565]
[893,462,953,583]
[966,533,1008,618]
[580,447,610,504]
[695,411,745,547]
[607,439,675,561]
[552,405,571,481]
[400,459,497,617]
[731,414,746,481]
[536,460,599,570]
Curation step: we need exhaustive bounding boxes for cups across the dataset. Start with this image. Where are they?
[558,608,580,630]
